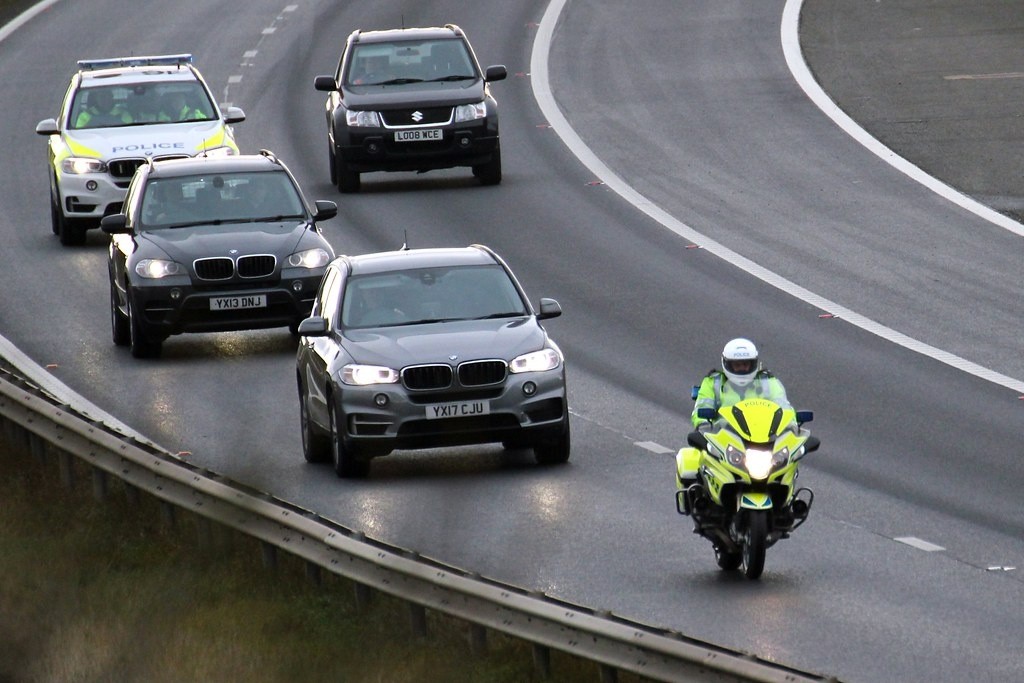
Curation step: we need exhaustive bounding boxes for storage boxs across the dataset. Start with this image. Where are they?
[677,445,703,511]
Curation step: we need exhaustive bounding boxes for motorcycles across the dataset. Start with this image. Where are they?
[675,386,821,580]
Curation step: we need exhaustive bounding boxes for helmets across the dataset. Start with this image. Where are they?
[720,337,759,387]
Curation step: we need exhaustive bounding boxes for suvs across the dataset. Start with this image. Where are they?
[36,53,246,247]
[101,148,339,358]
[296,244,570,477]
[315,23,507,193]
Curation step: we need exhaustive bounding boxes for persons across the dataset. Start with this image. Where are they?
[158,90,206,122]
[74,87,134,128]
[347,283,405,330]
[690,337,799,533]
[352,55,396,87]
[234,175,284,217]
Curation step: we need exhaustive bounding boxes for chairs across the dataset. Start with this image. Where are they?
[153,182,184,202]
[88,93,97,109]
